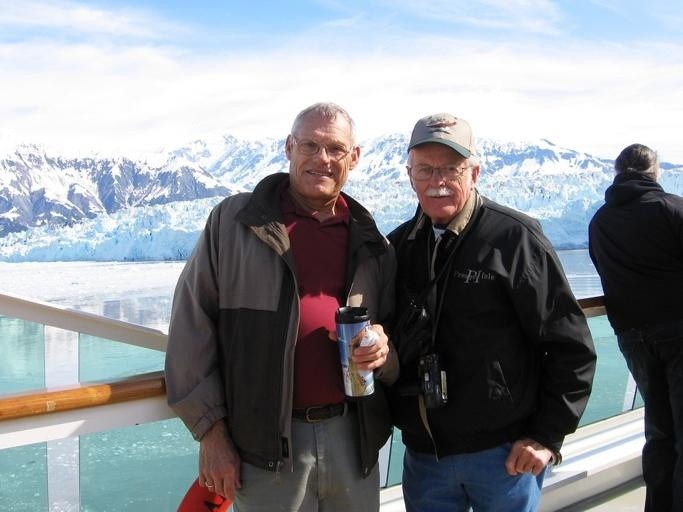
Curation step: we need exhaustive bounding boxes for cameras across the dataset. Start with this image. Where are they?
[417,353,448,409]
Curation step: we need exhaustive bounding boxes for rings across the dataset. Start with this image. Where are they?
[205,482,214,488]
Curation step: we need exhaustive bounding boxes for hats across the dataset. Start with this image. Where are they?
[408,116,473,157]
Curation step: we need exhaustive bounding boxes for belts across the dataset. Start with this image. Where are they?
[290,401,353,421]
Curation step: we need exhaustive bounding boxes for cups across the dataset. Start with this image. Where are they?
[334,306,374,399]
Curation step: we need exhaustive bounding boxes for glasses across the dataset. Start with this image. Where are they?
[408,164,470,179]
[290,131,354,158]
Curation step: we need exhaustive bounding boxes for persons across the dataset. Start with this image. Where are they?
[384,112,596,510]
[165,101,401,511]
[587,143,683,512]
